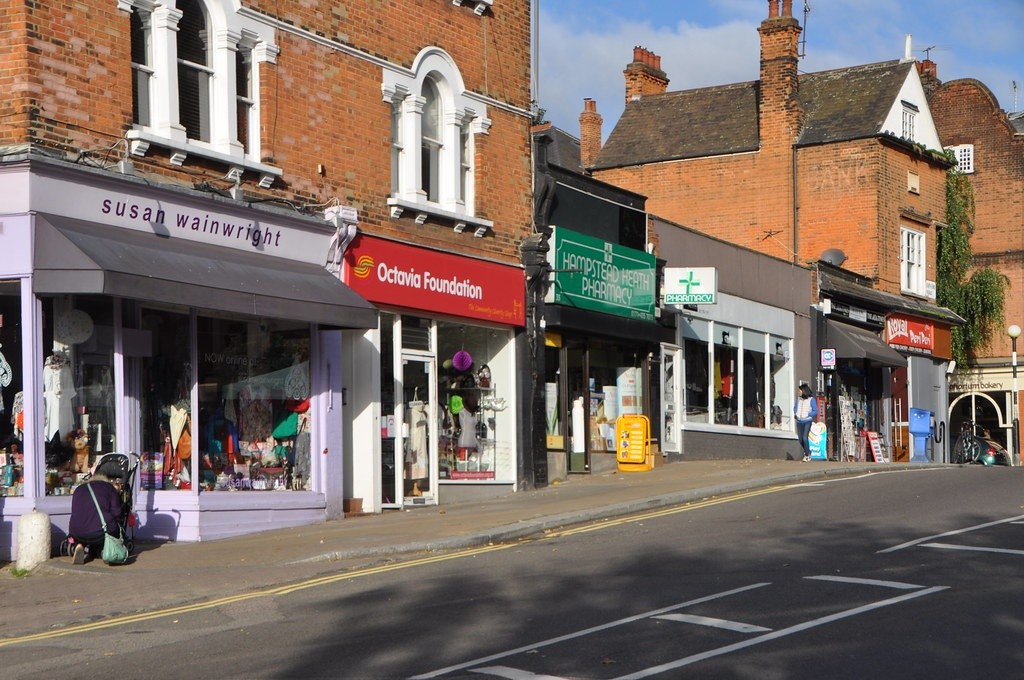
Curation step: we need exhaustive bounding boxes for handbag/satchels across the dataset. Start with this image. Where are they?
[103,532,130,564]
[285,397,311,412]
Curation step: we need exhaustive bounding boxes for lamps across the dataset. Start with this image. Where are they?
[80,138,134,175]
[302,197,343,228]
[201,168,243,201]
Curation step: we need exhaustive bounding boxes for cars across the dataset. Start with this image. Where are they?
[950,436,1011,467]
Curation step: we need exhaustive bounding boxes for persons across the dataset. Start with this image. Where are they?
[69,470,137,565]
[793,383,818,462]
[978,429,991,440]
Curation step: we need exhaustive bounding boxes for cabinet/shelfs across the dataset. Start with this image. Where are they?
[450,382,496,481]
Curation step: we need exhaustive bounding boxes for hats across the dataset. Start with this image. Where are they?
[463,394,478,413]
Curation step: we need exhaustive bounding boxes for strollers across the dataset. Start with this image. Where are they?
[59,446,141,557]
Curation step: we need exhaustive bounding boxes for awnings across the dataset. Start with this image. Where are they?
[31,208,378,334]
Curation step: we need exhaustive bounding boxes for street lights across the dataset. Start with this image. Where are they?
[1008,325,1021,470]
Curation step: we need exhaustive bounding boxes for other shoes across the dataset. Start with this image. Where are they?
[802,456,811,461]
[72,544,86,565]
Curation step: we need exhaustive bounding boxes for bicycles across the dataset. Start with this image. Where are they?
[952,418,985,464]
